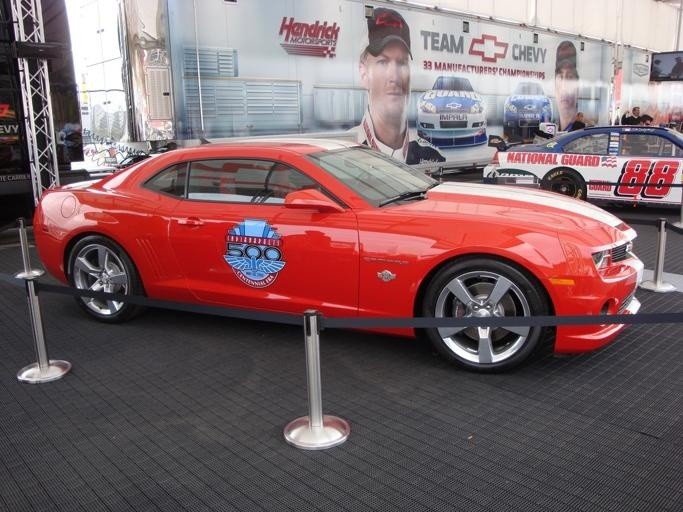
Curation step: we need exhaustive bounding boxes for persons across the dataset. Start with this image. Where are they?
[669,57,682,78]
[652,60,669,78]
[330,7,446,181]
[625,115,653,154]
[620,107,642,125]
[568,112,594,131]
[537,41,595,132]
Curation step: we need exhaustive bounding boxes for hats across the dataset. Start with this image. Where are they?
[554,40,578,78]
[359,7,412,63]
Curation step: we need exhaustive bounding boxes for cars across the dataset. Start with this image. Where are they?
[502,82,555,138]
[32,135,646,372]
[483,125,683,209]
[416,76,488,149]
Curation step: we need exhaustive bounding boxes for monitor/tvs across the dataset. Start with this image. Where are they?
[649,51,683,81]
